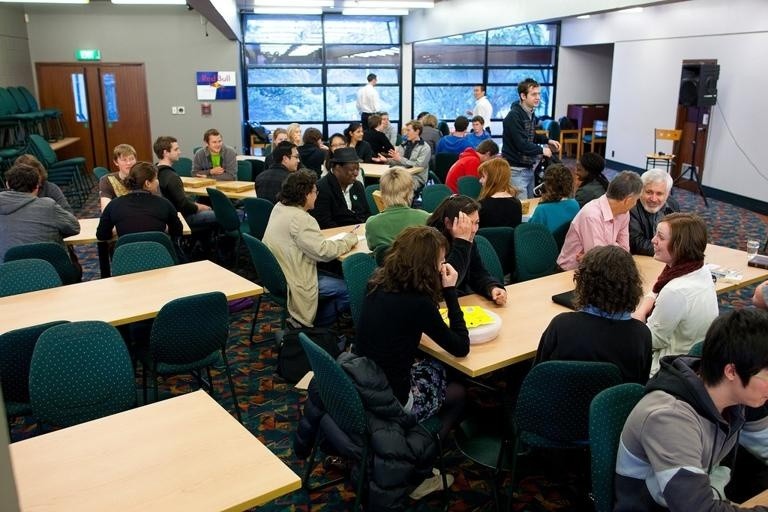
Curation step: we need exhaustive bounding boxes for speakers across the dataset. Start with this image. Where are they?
[680,64,720,108]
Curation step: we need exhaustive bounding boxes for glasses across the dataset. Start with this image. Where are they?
[309,188,320,197]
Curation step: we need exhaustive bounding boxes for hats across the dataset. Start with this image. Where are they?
[329,147,365,165]
[578,151,607,174]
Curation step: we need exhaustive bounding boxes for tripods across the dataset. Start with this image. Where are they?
[672,107,709,209]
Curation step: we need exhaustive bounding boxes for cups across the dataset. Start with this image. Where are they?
[747,240,760,260]
[520,201,530,214]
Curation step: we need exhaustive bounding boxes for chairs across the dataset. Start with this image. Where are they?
[2,83,764,512]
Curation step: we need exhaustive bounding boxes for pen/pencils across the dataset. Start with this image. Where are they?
[351,225,360,233]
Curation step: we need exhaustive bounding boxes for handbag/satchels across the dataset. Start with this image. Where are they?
[272,324,345,385]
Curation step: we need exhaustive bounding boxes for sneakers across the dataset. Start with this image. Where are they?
[408,466,457,501]
